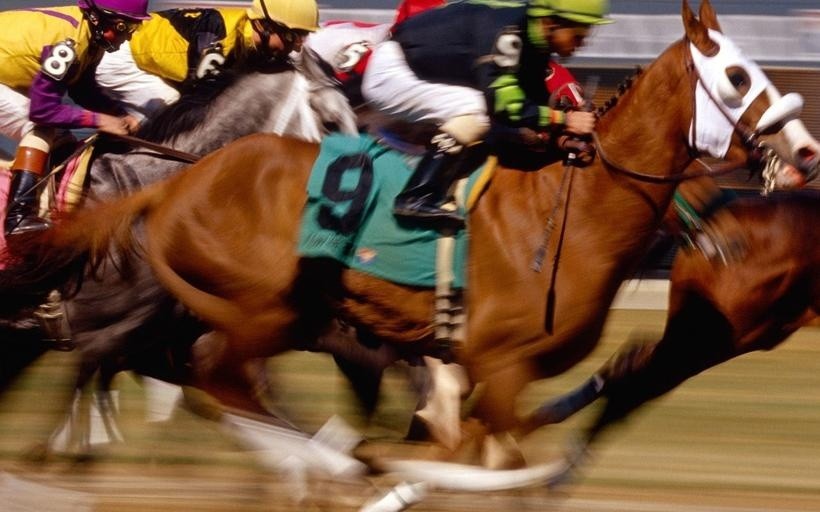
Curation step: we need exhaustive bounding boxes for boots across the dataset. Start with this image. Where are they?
[395,130,465,230]
[4,147,52,237]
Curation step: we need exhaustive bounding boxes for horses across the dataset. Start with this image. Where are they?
[0,0,820,512]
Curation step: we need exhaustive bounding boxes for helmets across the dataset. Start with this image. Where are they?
[80,1,151,19]
[399,2,444,23]
[532,0,616,23]
[246,0,318,32]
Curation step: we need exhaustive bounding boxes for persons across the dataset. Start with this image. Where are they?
[0,0,153,238]
[92,0,322,134]
[296,1,594,112]
[361,1,617,224]
